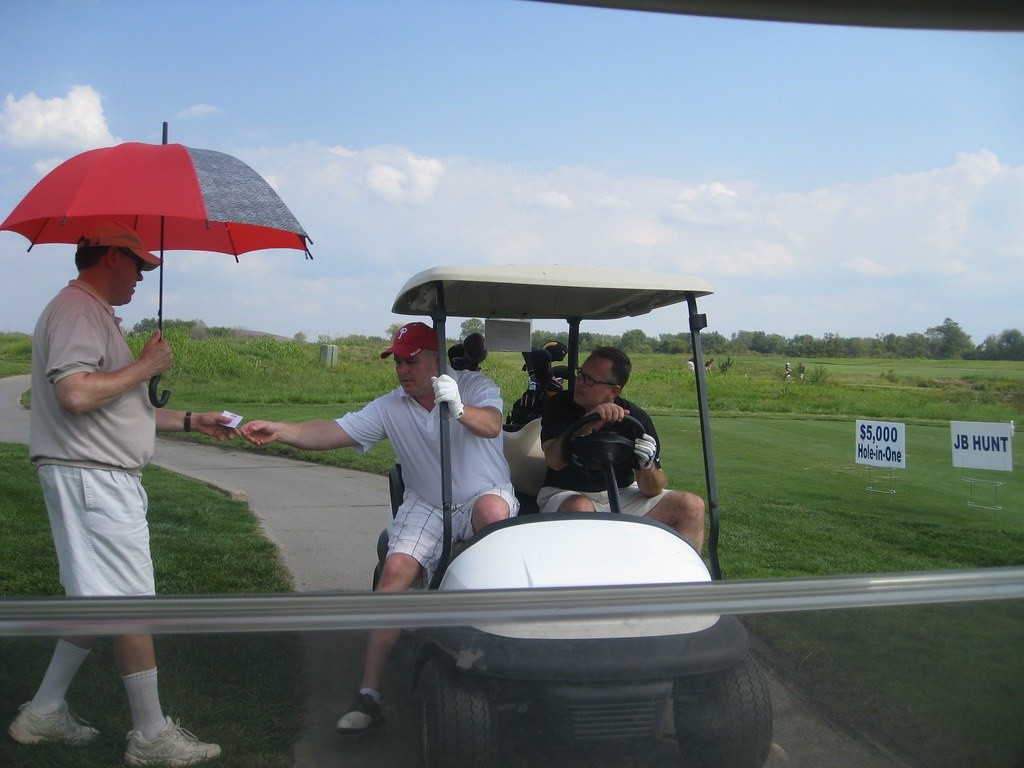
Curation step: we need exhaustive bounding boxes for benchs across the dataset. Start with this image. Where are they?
[503,417,545,516]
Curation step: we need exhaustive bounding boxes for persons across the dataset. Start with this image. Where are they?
[6,216,240,768]
[686,358,695,375]
[785,362,806,382]
[239,320,521,739]
[704,358,714,373]
[534,347,706,557]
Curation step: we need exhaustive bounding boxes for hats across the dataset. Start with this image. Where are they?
[74,220,164,271]
[381,322,439,358]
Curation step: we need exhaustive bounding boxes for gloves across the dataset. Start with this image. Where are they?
[634,433,657,470]
[431,374,464,420]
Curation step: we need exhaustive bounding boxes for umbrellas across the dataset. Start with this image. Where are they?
[1,121,313,407]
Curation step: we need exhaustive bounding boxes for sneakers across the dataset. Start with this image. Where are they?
[8,699,102,746]
[123,716,221,767]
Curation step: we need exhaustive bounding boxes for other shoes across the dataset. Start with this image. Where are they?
[335,689,386,735]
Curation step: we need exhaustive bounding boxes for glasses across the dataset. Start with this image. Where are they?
[574,367,616,387]
[119,248,144,274]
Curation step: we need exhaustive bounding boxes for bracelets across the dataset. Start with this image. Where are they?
[184,410,192,432]
